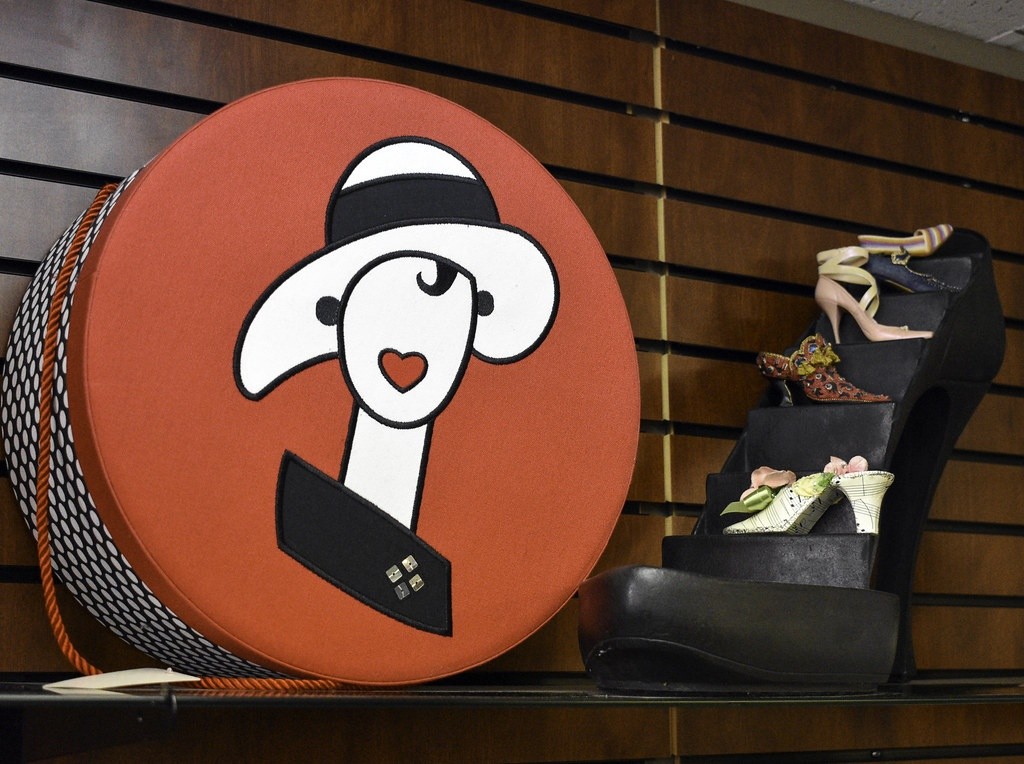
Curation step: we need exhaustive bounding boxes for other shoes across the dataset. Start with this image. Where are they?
[866,246,959,292]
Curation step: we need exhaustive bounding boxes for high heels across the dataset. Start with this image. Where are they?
[758,334,893,404]
[724,468,894,533]
[814,244,934,342]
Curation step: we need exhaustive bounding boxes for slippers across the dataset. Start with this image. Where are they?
[856,224,956,257]
